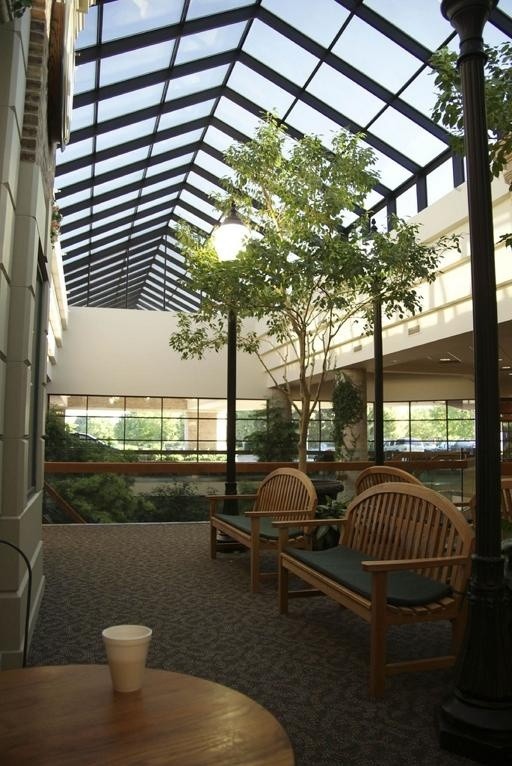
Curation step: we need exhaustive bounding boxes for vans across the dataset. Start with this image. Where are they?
[384,437,424,453]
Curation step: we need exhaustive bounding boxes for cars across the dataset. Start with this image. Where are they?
[308,442,335,458]
[70,431,118,451]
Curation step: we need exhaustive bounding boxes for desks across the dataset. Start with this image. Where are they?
[2,664,296,766]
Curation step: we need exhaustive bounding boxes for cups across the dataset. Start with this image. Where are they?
[101,625,151,694]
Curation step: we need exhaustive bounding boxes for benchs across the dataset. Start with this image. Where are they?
[354,465,473,524]
[467,476,511,526]
[271,482,478,688]
[209,465,317,588]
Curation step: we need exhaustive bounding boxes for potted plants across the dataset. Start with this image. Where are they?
[166,107,467,524]
